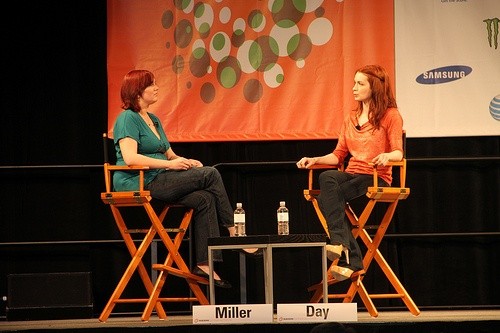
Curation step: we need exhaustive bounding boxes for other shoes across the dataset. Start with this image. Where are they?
[233,248,264,256]
[194,265,232,288]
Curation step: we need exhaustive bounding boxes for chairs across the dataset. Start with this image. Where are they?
[304,129,422,318]
[99,130,210,322]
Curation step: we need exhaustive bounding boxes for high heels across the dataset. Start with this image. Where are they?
[331,266,360,287]
[326,244,350,264]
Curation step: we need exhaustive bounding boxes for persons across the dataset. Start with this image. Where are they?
[296,64,404,287]
[112,67,239,290]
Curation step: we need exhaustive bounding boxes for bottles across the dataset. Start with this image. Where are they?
[276,201,289,235]
[234,203,245,237]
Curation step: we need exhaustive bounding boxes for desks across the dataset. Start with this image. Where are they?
[205,232,328,314]
